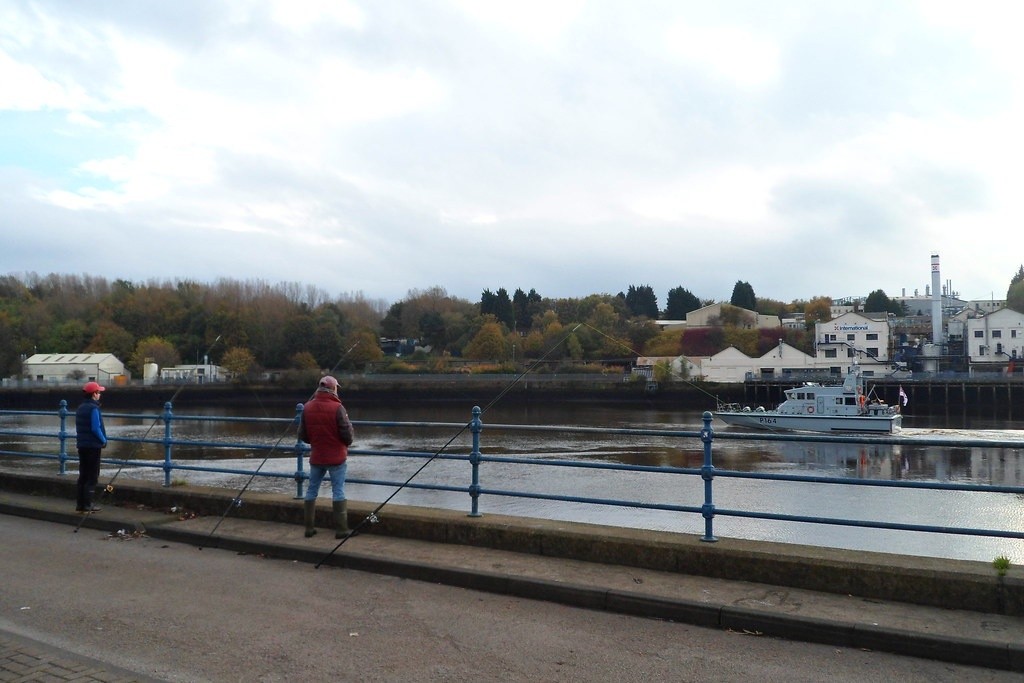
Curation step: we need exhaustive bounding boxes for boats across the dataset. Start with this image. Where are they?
[712,350,902,435]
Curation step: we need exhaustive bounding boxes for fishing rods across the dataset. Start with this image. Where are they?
[73,334,223,535]
[197,336,361,552]
[312,321,584,571]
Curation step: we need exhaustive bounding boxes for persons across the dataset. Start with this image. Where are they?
[297,377,359,539]
[75,382,107,511]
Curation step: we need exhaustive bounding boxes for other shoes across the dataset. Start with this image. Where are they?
[76,491,101,511]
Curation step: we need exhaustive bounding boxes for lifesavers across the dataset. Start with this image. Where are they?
[860,395,865,404]
[807,406,814,413]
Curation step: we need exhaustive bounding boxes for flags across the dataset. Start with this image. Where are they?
[900,387,908,406]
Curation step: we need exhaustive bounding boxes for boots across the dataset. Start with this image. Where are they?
[304,501,318,537]
[333,499,359,539]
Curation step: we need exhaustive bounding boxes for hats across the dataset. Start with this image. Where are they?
[319,376,341,388]
[81,382,105,394]
